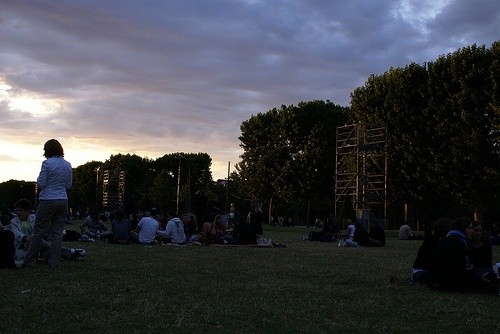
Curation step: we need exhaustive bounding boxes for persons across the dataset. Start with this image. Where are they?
[23,139,73,267]
[64,199,265,246]
[305,216,386,248]
[399,217,500,294]
[12,200,85,261]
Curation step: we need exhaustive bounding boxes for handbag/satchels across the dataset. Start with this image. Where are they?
[258,237,272,248]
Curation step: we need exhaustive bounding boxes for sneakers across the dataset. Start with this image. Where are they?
[70,248,86,257]
